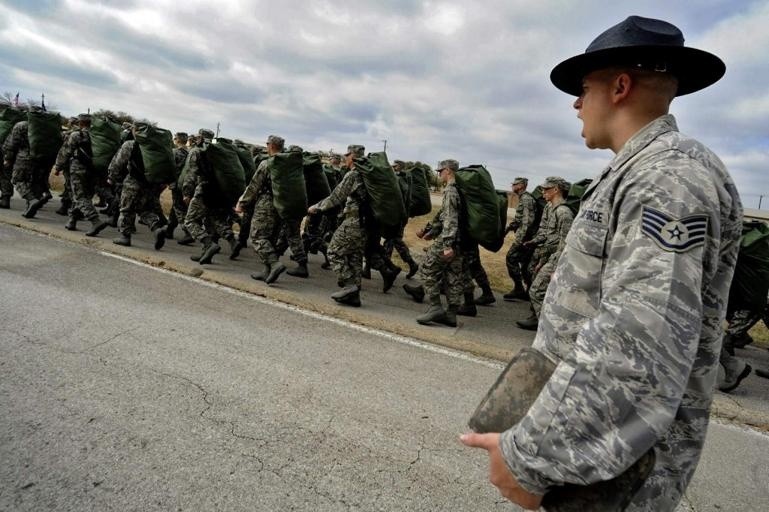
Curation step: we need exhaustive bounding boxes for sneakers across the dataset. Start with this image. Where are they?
[504,286,538,330]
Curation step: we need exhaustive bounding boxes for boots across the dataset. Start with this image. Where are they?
[252,255,308,282]
[402,280,495,326]
[719,350,752,391]
[320,251,418,306]
[0,190,241,264]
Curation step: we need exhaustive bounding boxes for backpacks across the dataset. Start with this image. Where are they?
[353,152,407,226]
[269,153,336,220]
[530,186,548,207]
[458,165,507,252]
[1,106,62,156]
[406,167,431,217]
[90,119,121,180]
[200,142,256,193]
[135,127,199,188]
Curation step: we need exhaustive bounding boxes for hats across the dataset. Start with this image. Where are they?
[288,145,303,152]
[511,178,528,184]
[435,160,459,172]
[540,178,571,192]
[68,115,187,141]
[344,145,365,156]
[550,17,726,97]
[267,135,284,147]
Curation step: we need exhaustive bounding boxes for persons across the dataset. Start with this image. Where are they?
[716,262,769,392]
[501,174,575,330]
[458,14,745,511]
[401,159,495,326]
[0,100,419,308]
